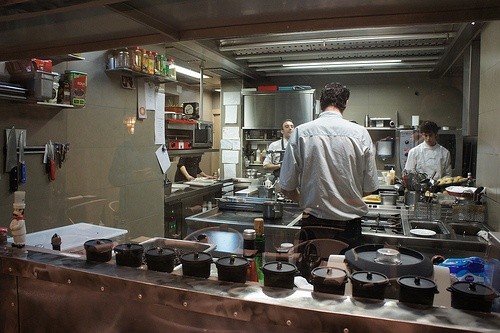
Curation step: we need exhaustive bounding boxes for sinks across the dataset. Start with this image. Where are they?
[7,223,128,251]
[409,219,450,238]
[449,223,488,236]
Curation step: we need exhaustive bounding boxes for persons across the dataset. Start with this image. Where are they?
[404,120,452,180]
[278,81,379,254]
[9,192,27,248]
[174,156,217,182]
[263,118,295,181]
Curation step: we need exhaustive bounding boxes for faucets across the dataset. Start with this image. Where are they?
[34,244,44,248]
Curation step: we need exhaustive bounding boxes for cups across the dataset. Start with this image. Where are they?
[406,191,418,206]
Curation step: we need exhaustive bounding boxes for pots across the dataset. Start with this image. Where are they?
[349,270,392,300]
[214,256,251,283]
[393,275,439,305]
[83,239,113,262]
[114,243,144,267]
[144,247,179,274]
[259,261,301,290]
[263,203,284,220]
[306,266,349,296]
[179,251,213,278]
[446,281,500,313]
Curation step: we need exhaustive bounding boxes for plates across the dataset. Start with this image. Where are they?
[410,229,436,236]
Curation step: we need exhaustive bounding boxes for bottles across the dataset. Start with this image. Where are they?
[254,218,266,285]
[262,149,266,164]
[106,48,129,69]
[256,148,261,162]
[202,202,212,213]
[153,52,177,80]
[390,168,395,185]
[465,172,474,187]
[243,229,258,282]
[130,46,154,75]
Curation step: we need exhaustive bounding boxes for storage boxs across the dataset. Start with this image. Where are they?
[10,70,58,102]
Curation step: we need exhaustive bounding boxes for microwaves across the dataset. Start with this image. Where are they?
[191,120,213,148]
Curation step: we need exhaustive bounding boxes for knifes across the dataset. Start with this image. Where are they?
[6,125,57,192]
